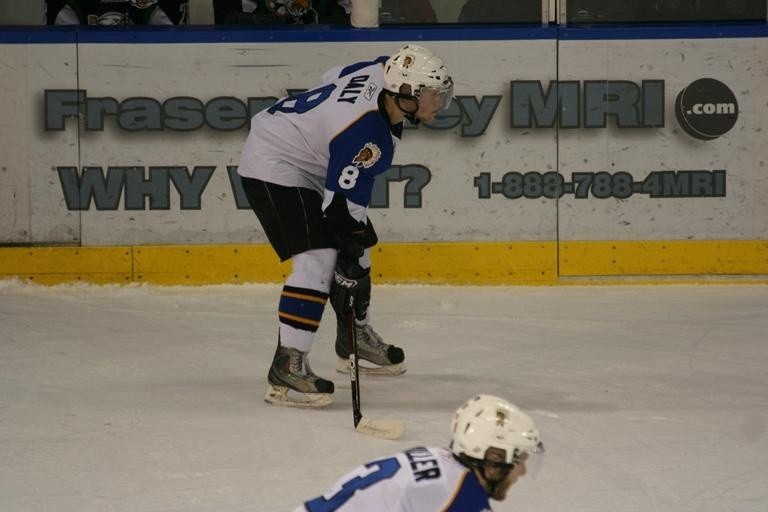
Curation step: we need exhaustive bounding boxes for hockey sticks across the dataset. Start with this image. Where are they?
[348,296,403,439]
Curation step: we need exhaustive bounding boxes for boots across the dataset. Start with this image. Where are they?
[335,315,404,365]
[268,327,334,394]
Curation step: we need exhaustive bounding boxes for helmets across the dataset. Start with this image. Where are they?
[451,394,544,466]
[384,44,453,111]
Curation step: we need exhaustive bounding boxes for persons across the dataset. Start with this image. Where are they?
[290,391,548,512]
[214,1,766,29]
[41,1,189,28]
[235,43,458,396]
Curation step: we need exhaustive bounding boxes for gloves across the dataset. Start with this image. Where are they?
[330,261,370,326]
